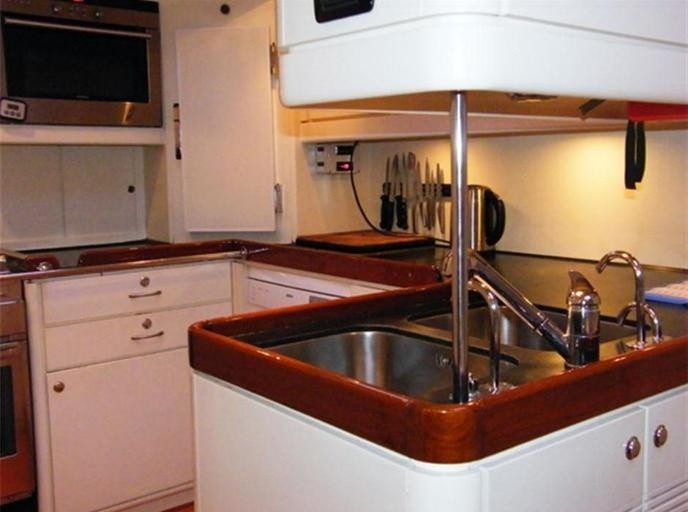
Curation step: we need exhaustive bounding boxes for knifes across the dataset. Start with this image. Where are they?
[377,152,446,237]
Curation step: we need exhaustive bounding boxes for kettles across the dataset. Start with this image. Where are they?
[449,183,506,264]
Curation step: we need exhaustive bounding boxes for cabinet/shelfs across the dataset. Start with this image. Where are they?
[0,145,146,252]
[42,261,234,512]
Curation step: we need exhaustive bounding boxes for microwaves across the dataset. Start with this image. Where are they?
[0,1,167,130]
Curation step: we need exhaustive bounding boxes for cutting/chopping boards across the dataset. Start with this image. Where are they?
[294,223,435,256]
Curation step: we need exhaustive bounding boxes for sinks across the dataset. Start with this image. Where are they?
[256,328,517,396]
[408,302,651,351]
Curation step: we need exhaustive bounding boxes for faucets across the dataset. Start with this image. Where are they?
[450,277,503,390]
[596,251,647,348]
[442,245,600,366]
[616,301,663,342]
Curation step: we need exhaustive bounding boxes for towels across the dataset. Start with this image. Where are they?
[645,280,688,306]
